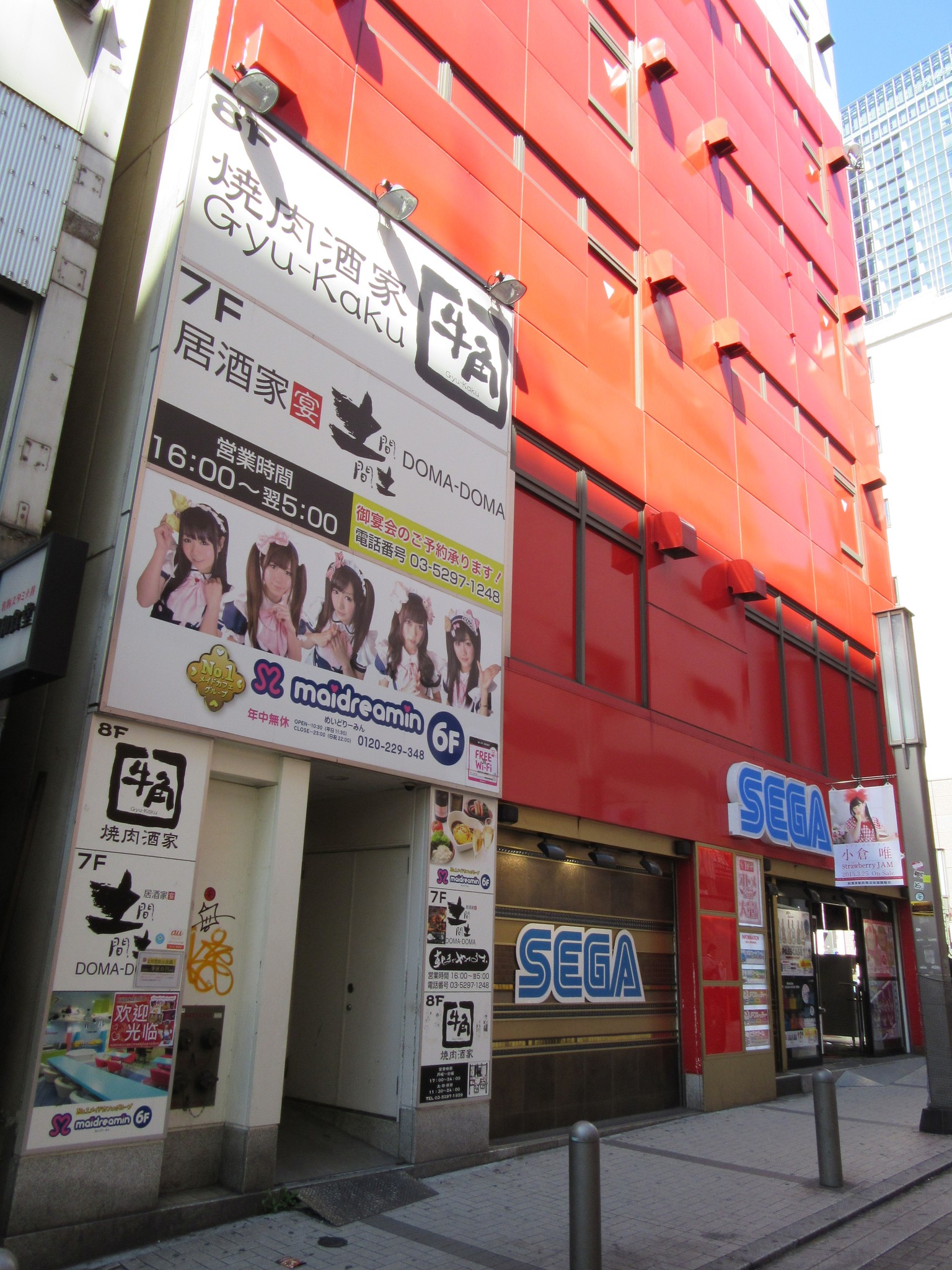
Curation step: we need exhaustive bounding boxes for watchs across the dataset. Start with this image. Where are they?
[479,702,488,708]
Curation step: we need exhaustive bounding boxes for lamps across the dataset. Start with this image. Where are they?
[210,56,528,311]
[536,831,667,878]
[763,873,889,913]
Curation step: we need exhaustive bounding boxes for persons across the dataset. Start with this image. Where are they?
[844,788,898,843]
[298,551,377,680]
[137,489,238,637]
[375,580,447,704]
[442,608,501,717]
[221,525,306,661]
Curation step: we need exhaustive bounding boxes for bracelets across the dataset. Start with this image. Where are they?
[400,688,403,692]
